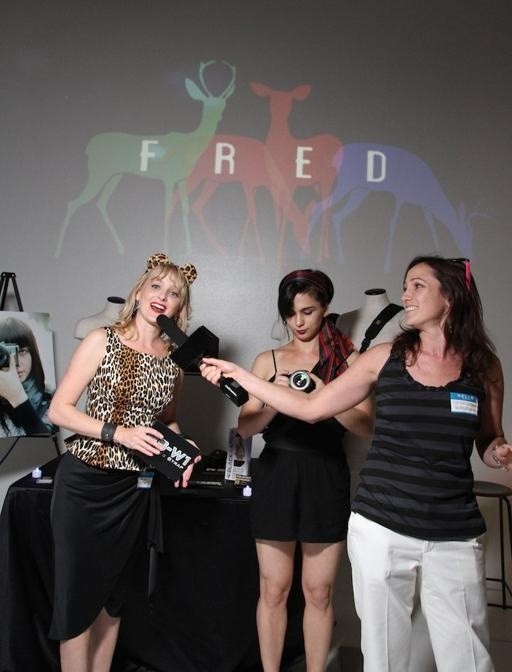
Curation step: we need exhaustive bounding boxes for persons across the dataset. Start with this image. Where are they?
[234,270,374,671]
[47,254,189,671]
[0,315,55,436]
[200,257,512,672]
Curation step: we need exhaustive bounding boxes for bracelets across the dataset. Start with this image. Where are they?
[102,421,117,446]
[491,443,500,467]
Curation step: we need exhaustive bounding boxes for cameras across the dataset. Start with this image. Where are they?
[0,341,19,368]
[284,369,315,393]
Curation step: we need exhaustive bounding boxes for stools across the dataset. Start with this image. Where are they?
[473,480,512,610]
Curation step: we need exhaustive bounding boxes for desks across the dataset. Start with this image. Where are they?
[1,449,305,672]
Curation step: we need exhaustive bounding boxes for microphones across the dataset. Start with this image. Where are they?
[156,315,249,407]
